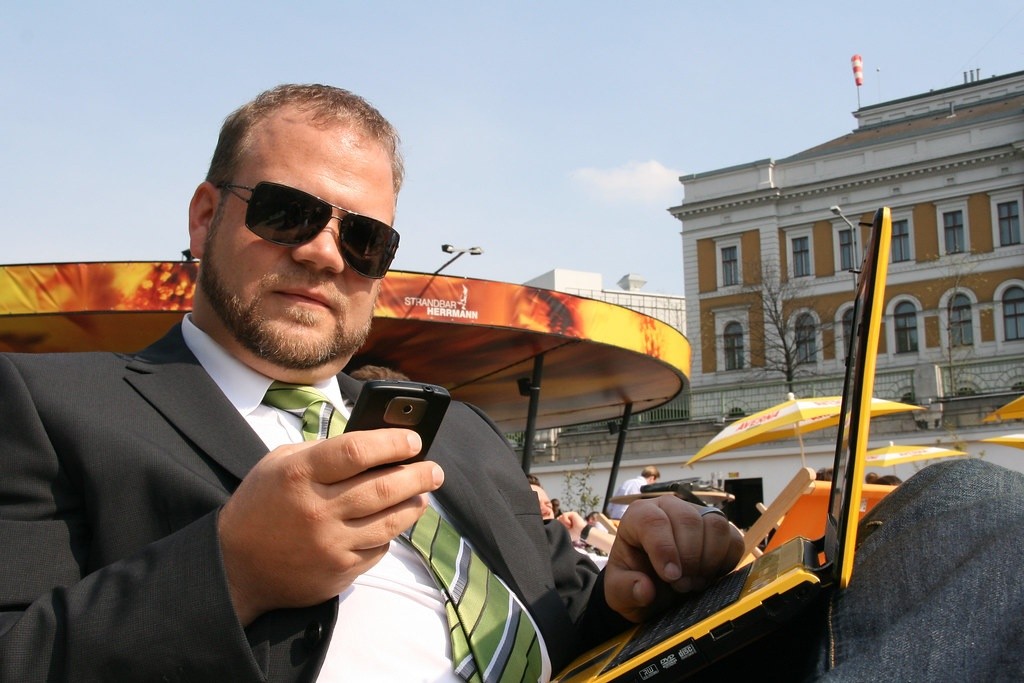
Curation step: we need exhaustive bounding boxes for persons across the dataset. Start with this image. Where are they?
[524,465,666,571]
[2,80,1024,682]
[812,467,905,488]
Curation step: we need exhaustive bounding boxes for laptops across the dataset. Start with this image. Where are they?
[545,207,892,683]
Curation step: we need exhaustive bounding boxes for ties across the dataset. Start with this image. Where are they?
[263,380,542,683]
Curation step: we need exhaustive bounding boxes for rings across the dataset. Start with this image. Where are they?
[697,506,728,523]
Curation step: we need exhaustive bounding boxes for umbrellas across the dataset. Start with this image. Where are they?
[861,389,1024,484]
[682,390,929,475]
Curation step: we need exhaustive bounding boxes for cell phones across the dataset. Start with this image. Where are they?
[344,381,450,479]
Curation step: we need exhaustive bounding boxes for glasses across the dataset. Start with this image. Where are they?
[220,180,401,280]
